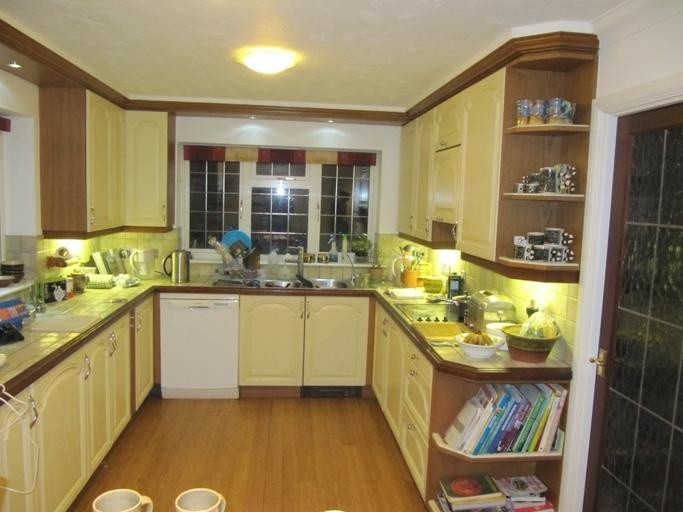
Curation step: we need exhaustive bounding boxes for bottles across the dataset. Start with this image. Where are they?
[358,272,370,288]
[341,236,347,255]
[60,270,84,299]
[304,253,328,263]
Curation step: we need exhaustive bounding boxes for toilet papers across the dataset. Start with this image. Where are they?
[65,257,80,267]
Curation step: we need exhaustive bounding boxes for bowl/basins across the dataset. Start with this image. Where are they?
[0,262,24,287]
[456,322,560,362]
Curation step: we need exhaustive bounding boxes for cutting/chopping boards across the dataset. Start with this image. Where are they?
[410,322,474,340]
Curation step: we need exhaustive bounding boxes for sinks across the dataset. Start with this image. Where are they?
[259,279,296,289]
[297,278,353,290]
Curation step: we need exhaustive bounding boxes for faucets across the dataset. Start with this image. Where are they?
[342,253,363,288]
[287,245,304,280]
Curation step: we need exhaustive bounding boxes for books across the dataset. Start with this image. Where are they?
[436,380,569,512]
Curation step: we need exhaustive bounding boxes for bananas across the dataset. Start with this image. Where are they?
[463,330,493,346]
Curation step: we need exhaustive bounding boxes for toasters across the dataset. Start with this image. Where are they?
[466,290,515,335]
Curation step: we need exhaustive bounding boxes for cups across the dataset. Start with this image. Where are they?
[512,97,577,263]
[400,270,417,288]
[91,488,154,511]
[175,488,226,511]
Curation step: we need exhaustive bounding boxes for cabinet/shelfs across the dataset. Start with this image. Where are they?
[494,52,593,283]
[122,109,175,234]
[396,105,455,250]
[39,87,122,239]
[370,300,403,445]
[239,293,370,397]
[449,67,505,270]
[87,311,132,478]
[0,385,43,512]
[42,346,91,511]
[433,89,463,152]
[399,336,433,501]
[131,294,154,413]
[423,370,568,512]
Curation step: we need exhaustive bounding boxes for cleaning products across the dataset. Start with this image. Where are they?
[327,233,339,262]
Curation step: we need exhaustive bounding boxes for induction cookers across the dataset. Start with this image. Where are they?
[394,301,467,323]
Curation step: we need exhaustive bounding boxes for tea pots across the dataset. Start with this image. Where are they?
[129,247,155,280]
[162,249,193,283]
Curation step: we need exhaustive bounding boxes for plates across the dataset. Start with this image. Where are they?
[220,230,251,249]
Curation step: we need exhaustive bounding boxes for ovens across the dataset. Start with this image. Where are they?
[160,292,241,400]
